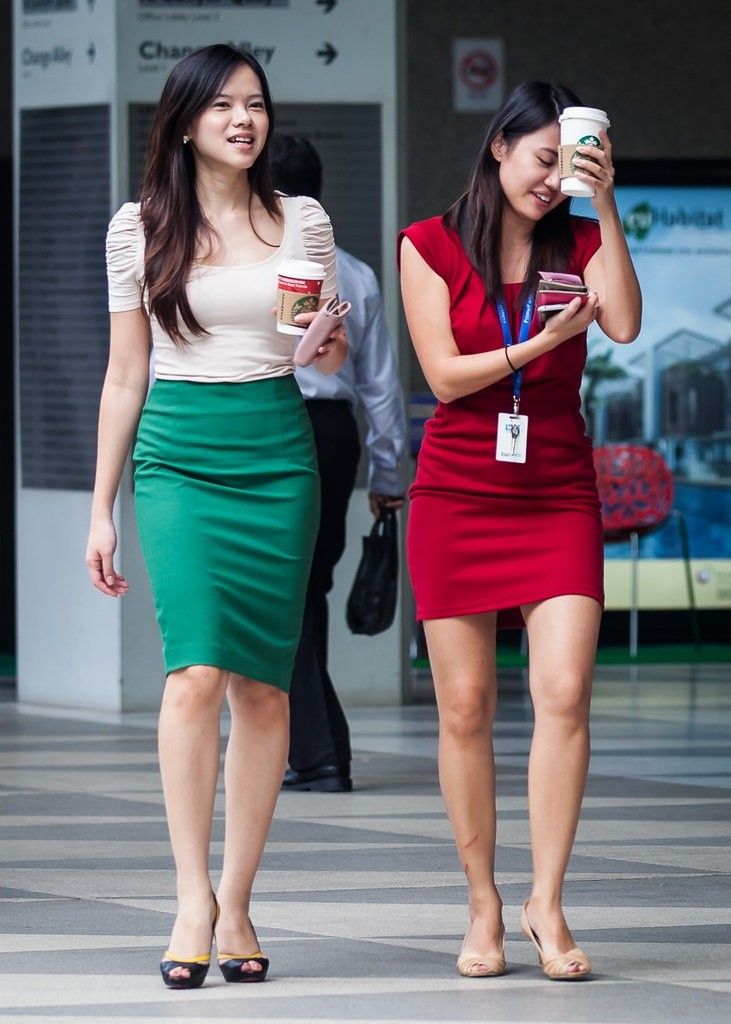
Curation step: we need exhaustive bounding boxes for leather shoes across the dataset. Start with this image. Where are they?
[278,767,352,793]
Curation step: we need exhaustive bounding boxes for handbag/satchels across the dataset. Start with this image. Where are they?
[347,506,397,634]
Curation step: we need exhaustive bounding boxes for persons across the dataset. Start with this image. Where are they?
[393,80,643,980]
[252,133,405,794]
[83,44,348,990]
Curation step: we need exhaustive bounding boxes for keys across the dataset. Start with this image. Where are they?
[511,424,520,455]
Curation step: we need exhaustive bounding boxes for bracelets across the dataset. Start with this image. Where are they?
[505,345,515,372]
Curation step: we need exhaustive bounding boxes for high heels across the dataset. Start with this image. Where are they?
[213,913,269,982]
[521,901,590,977]
[457,920,505,978]
[161,891,221,988]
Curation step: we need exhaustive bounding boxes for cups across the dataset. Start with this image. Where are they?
[276,259,327,337]
[557,105,611,197]
